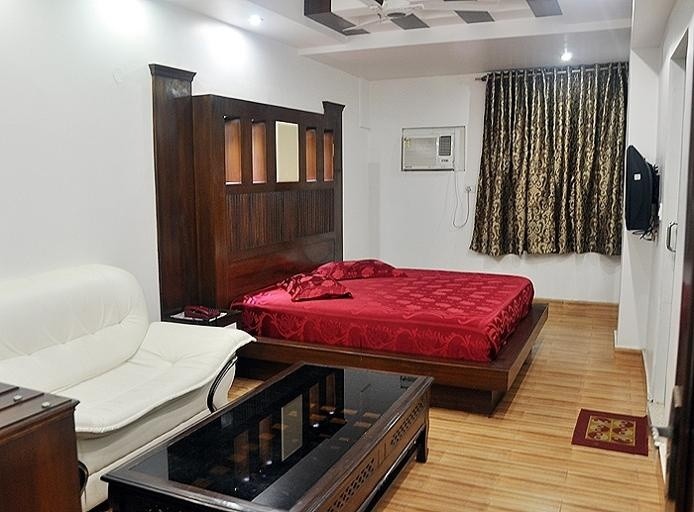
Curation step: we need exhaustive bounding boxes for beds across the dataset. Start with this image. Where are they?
[230,261,549,415]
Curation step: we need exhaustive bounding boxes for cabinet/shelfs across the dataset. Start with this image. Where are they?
[191,94,345,311]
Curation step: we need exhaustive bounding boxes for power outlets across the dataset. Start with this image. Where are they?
[465,183,476,192]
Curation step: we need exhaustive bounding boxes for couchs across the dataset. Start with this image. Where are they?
[0,264,256,512]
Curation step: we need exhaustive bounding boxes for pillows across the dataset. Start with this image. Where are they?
[277,273,354,301]
[313,260,408,279]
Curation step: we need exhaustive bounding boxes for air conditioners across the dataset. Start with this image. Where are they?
[403,134,456,171]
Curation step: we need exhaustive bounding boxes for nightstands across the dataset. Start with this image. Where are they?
[164,310,242,330]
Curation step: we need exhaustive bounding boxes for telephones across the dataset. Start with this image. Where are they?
[185,306,220,319]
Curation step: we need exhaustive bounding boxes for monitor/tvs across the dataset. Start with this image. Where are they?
[623,145,658,231]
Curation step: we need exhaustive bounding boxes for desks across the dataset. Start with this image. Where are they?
[0,383,84,512]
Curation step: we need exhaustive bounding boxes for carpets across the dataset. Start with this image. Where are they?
[571,408,649,457]
